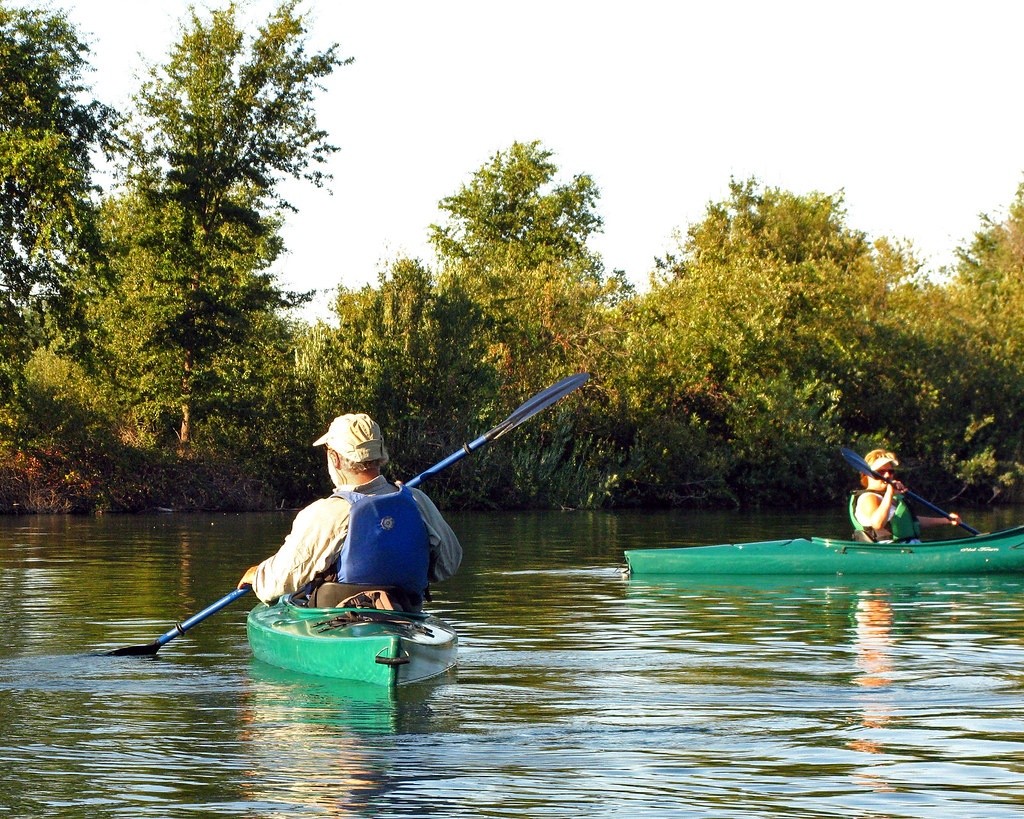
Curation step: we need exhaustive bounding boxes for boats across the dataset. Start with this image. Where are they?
[246,568,460,689]
[624,524,1024,575]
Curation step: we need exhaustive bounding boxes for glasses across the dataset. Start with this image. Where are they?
[874,468,895,478]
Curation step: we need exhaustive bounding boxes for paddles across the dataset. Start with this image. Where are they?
[836,438,981,534]
[106,366,587,656]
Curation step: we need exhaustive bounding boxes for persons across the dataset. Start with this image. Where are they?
[238,413,463,608]
[849,449,963,544]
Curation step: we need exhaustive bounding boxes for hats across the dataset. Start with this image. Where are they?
[869,458,898,471]
[312,413,384,462]
